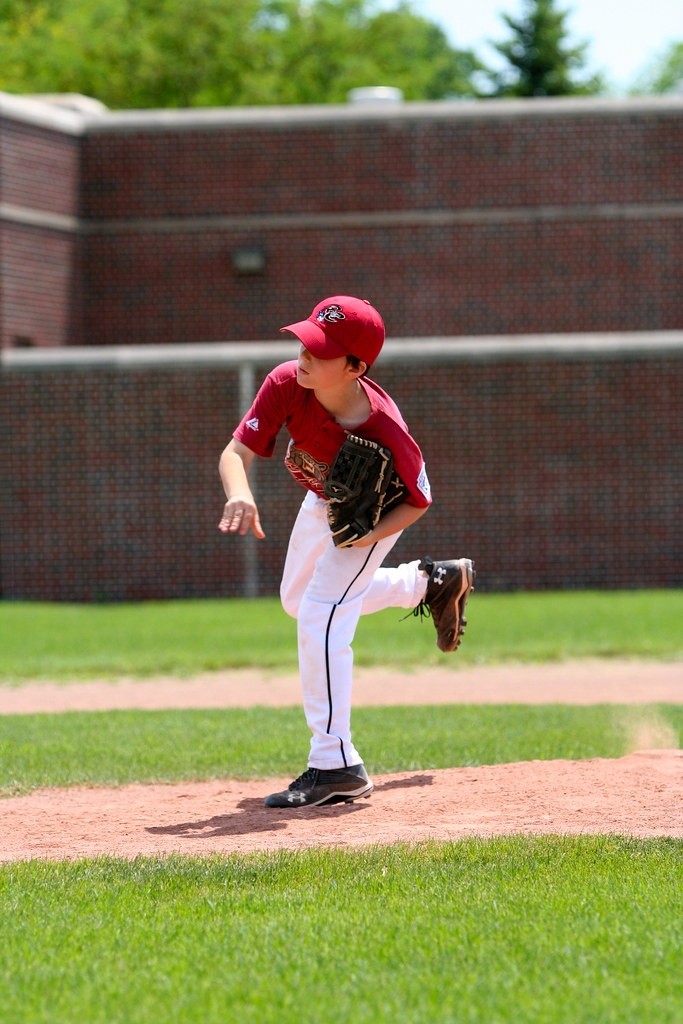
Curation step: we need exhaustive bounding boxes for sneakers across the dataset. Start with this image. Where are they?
[398,556,476,652]
[266,761,374,808]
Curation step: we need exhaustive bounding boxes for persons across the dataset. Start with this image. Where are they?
[217,295,477,809]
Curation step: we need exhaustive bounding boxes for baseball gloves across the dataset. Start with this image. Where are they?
[320,432,413,550]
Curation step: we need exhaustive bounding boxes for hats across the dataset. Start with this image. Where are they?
[280,295,387,367]
